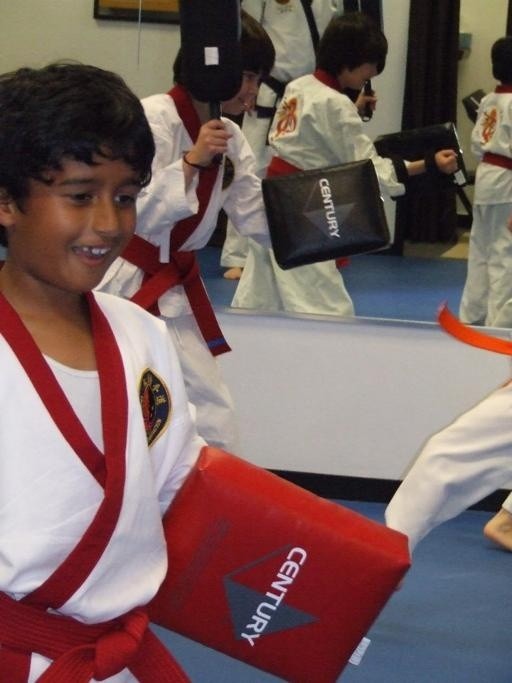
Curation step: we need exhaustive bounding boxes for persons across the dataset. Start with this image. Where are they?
[219,1,460,321]
[385,380,512,591]
[1,65,408,683]
[460,36,512,325]
[92,0,393,320]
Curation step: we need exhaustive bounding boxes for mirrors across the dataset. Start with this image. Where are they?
[0,0,511,333]
